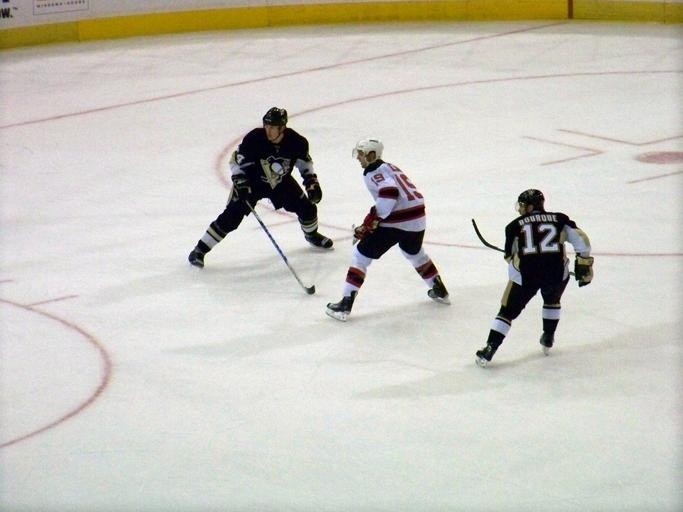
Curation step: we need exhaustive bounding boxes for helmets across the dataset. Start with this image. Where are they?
[352,138,384,168]
[263,106,289,126]
[518,189,545,206]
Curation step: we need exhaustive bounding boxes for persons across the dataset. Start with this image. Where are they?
[474,187,595,365]
[323,139,450,319]
[185,107,333,268]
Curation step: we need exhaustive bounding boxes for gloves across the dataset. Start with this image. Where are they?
[574,255,595,288]
[305,182,322,204]
[231,172,252,200]
[353,206,383,240]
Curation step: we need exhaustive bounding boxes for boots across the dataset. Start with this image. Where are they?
[427,274,448,298]
[326,289,358,313]
[475,341,498,361]
[304,225,333,248]
[540,331,553,347]
[188,246,206,268]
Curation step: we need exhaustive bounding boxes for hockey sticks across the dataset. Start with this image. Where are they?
[471,218,576,278]
[244,198,317,296]
